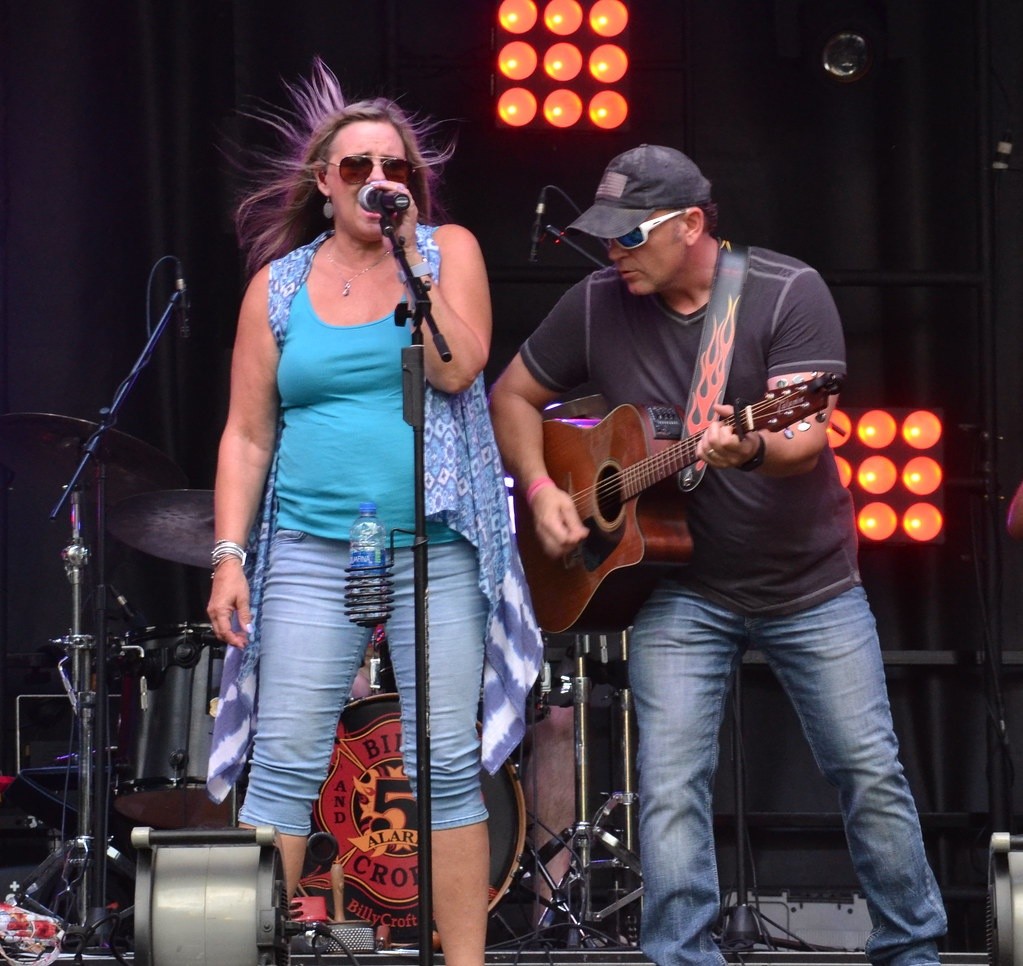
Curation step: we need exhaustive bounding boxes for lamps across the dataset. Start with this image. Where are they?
[823,404,954,549]
[487,0,637,143]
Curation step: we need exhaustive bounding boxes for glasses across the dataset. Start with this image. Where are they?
[600,210,685,249]
[315,155,417,186]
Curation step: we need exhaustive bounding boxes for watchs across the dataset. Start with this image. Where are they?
[734,435,766,473]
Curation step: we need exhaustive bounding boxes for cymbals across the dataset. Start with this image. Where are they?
[106,488,214,569]
[1,412,188,508]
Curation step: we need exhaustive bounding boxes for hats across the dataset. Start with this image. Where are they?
[564,143,712,240]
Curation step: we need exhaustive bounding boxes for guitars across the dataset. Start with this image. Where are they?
[514,372,841,638]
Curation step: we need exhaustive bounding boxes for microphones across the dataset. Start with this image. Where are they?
[174,261,191,340]
[110,585,149,631]
[529,189,548,263]
[357,184,410,213]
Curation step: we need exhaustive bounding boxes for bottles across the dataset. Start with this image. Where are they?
[349,502,387,619]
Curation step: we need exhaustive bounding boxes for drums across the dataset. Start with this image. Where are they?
[109,623,257,829]
[245,693,528,948]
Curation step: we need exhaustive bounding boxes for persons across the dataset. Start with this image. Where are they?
[489,142,948,966]
[204,55,545,966]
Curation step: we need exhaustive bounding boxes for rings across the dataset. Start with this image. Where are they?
[705,449,715,457]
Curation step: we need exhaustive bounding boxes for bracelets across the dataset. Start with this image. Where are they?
[209,540,246,578]
[527,476,556,508]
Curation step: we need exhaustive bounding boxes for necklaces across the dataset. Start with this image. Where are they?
[326,245,394,297]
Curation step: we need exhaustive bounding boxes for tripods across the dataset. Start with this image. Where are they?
[7,292,181,948]
[513,630,849,951]
